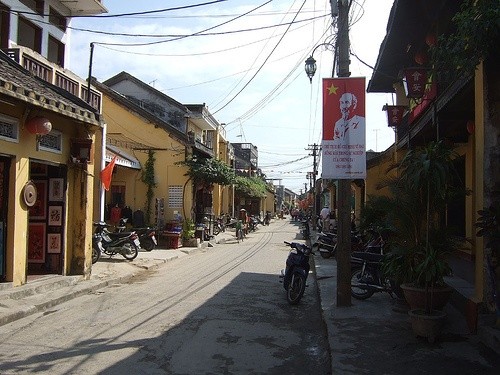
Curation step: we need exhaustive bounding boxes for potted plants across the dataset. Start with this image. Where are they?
[363,147,472,337]
[183,219,200,247]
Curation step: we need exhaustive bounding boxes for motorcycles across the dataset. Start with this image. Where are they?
[92,221,142,265]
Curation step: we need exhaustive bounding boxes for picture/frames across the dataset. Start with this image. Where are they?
[28,222,47,264]
[29,178,48,222]
[47,203,64,227]
[47,232,62,254]
[48,176,65,203]
[31,162,49,178]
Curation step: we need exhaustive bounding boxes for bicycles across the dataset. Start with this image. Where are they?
[195,206,288,244]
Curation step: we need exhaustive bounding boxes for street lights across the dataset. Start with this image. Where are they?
[304,42,352,308]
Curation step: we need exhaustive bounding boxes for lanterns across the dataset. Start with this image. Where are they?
[25,117,52,136]
[415,52,427,64]
[425,31,437,46]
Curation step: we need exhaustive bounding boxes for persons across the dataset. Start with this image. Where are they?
[219,200,337,232]
[334,93,365,140]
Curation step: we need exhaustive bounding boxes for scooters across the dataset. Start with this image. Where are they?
[114,217,158,252]
[293,208,384,259]
[350,242,405,301]
[279,241,316,306]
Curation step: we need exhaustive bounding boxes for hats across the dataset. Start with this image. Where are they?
[323,204,329,208]
